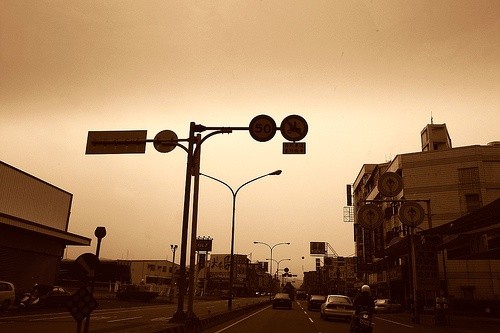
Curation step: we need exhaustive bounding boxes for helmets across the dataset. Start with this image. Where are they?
[361,285,370,293]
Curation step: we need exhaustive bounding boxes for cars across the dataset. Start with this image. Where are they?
[320,295,355,319]
[272,294,293,308]
[307,295,326,312]
[38,286,71,307]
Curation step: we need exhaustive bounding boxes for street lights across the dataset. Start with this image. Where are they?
[192,170,283,312]
[252,241,292,299]
[170,244,179,287]
[265,258,291,283]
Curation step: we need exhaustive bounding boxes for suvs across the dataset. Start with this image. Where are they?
[0,281,16,310]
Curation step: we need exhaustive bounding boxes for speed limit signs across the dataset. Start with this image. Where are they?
[248,115,277,142]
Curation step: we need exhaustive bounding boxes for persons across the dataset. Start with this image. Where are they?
[436,280,451,321]
[25,283,41,306]
[353,284,375,317]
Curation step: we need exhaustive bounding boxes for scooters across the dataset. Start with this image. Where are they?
[18,289,41,308]
[350,307,377,333]
